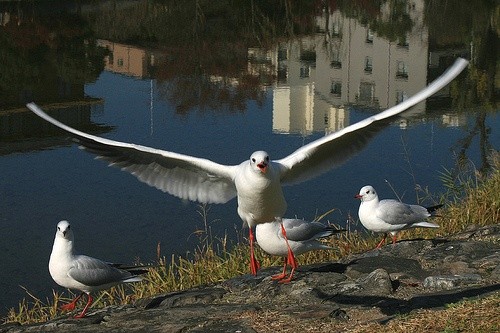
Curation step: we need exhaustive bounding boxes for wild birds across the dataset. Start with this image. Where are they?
[28,56,470,276]
[354,186,444,249]
[48,220,149,319]
[254,218,347,286]
